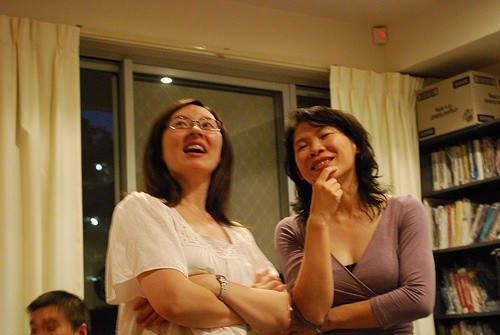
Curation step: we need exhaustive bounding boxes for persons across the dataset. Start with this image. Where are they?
[274,105,435,335]
[105,98,295,335]
[26,289,90,335]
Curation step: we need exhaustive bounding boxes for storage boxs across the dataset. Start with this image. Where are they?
[416,70,500,142]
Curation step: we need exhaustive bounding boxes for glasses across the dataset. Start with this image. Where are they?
[166,115,223,132]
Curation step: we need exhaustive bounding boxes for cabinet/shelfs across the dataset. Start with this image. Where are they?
[419,117,500,335]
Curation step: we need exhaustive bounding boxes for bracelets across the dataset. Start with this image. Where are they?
[216,275,227,301]
[316,327,322,335]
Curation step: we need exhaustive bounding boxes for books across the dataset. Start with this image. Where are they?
[423,139,500,335]
